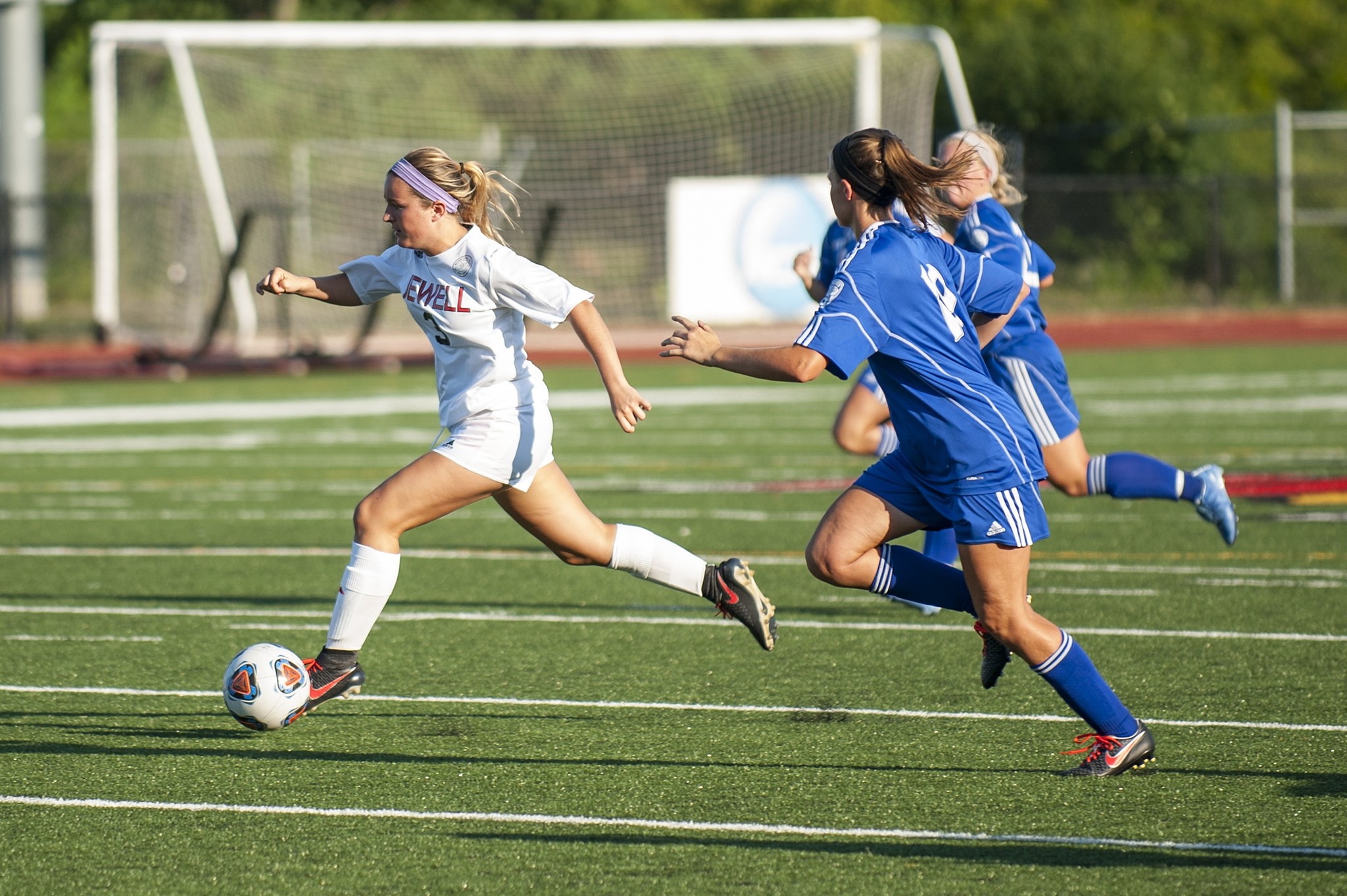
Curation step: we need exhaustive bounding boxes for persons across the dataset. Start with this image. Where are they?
[793,196,961,459]
[660,128,1156,779]
[890,130,1240,617]
[257,146,780,719]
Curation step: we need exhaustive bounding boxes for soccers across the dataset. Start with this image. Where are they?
[221,642,312,733]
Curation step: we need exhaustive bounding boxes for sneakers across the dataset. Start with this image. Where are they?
[302,644,367,715]
[701,558,779,652]
[1059,719,1156,778]
[1191,463,1239,547]
[973,593,1032,688]
